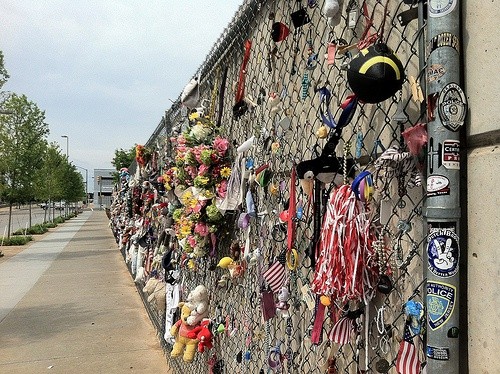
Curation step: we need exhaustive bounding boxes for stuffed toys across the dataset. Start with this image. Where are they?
[107,168,158,252]
[297,137,356,196]
[324,0,343,26]
[133,266,166,312]
[167,285,214,364]
[218,257,235,270]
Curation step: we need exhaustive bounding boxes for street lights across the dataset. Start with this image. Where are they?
[61,135,69,216]
[73,165,88,207]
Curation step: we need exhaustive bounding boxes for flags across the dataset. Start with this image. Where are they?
[396,314,423,374]
[263,251,286,295]
[141,237,154,278]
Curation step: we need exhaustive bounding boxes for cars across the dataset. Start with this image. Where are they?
[40,201,81,210]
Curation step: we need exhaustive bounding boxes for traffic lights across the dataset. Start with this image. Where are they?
[97,176,100,182]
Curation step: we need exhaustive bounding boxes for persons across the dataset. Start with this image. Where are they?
[101,200,118,211]
[81,204,86,210]
[90,201,94,212]
